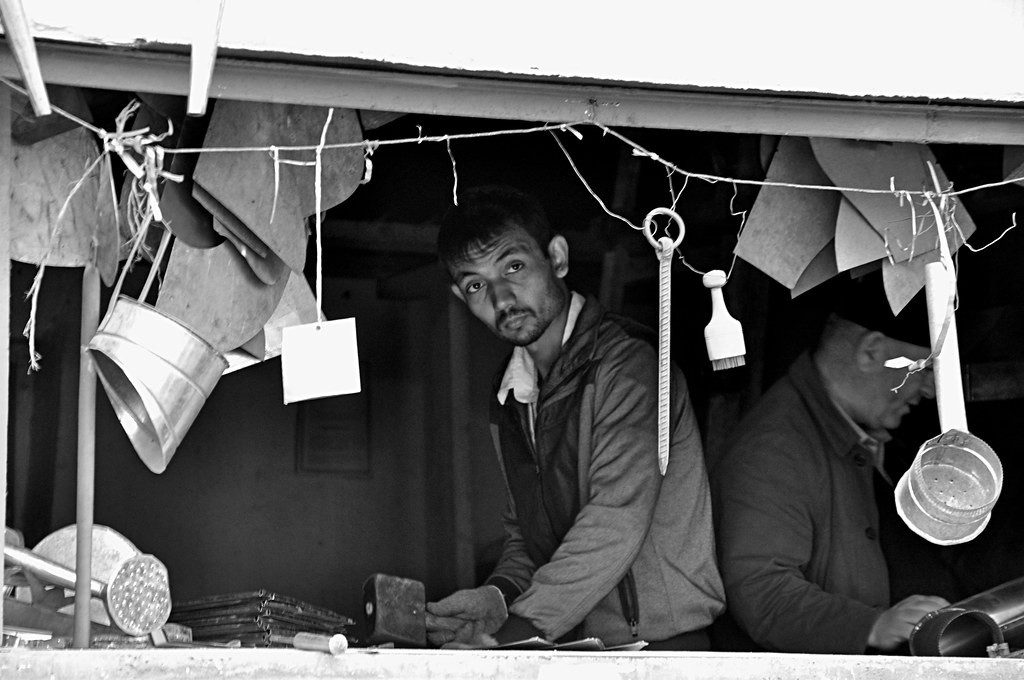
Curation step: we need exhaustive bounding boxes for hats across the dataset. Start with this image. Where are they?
[832,266,932,348]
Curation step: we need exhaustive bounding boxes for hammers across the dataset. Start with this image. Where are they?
[361,573,467,649]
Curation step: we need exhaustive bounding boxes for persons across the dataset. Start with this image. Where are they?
[426,187,726,650]
[708,275,952,651]
